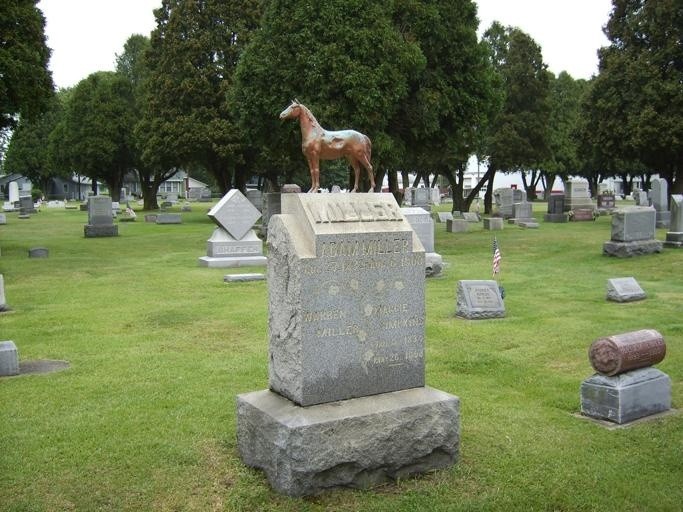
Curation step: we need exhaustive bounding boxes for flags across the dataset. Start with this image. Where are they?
[126,202,136,220]
[493,238,501,276]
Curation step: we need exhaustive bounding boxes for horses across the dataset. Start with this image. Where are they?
[280,98,376,193]
